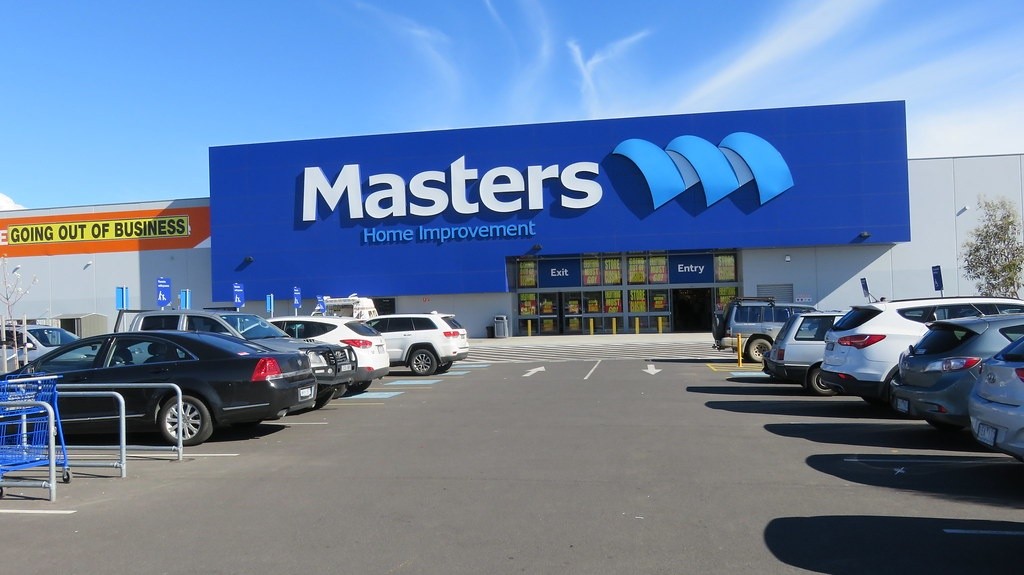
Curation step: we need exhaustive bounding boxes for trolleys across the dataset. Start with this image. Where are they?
[0,372,72,498]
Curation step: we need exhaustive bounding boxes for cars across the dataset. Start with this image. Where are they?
[968,336,1024,462]
[889,313,1024,430]
[762,309,851,396]
[0,324,99,361]
[239,316,390,396]
[365,311,469,376]
[0,330,317,447]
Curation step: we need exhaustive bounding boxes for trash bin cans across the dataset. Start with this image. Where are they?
[494,315,509,338]
[486,326,494,338]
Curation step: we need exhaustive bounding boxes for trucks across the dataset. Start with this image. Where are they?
[114,309,358,410]
[310,293,378,318]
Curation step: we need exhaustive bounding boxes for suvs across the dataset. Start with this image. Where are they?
[820,297,1024,404]
[712,296,820,363]
